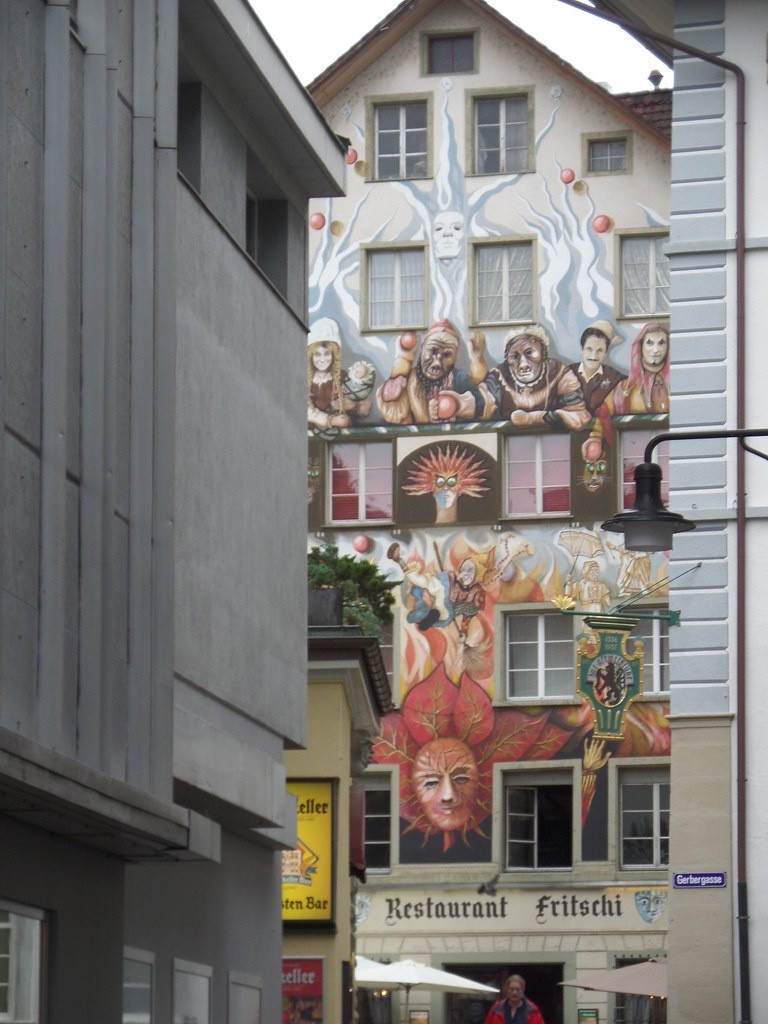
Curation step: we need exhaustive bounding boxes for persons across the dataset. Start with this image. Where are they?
[485,974,545,1024]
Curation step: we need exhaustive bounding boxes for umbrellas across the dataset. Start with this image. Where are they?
[557,957,667,999]
[355,955,500,1024]
